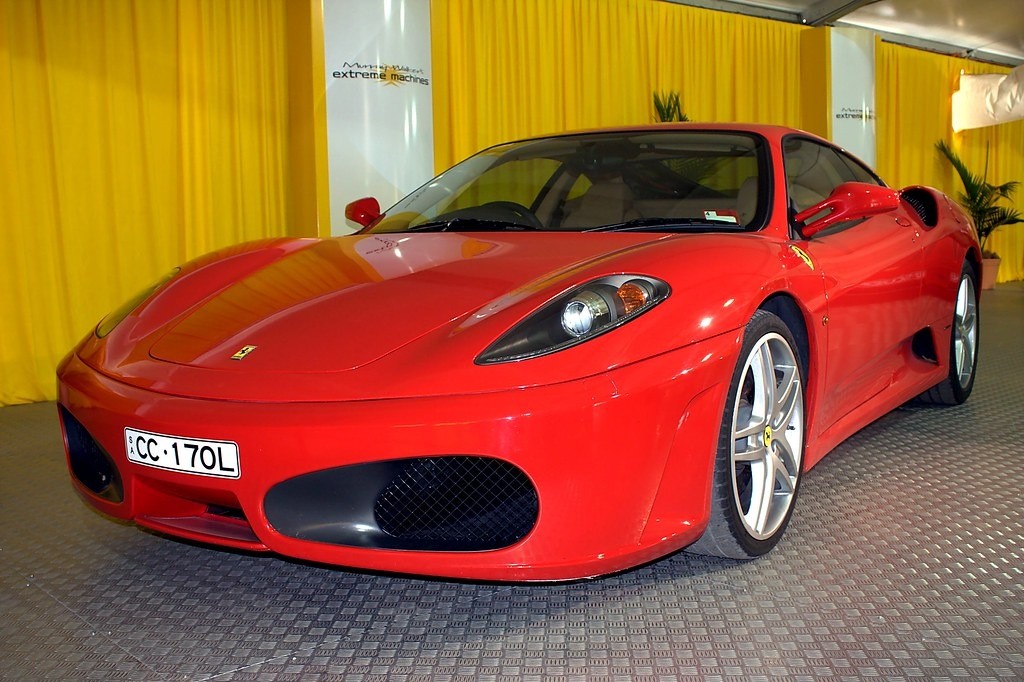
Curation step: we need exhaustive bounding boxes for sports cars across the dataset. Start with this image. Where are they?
[55,126,982,584]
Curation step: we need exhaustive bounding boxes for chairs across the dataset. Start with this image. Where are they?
[737,177,793,225]
[558,180,631,230]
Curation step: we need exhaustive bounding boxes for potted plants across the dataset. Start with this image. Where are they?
[934,139,1024,290]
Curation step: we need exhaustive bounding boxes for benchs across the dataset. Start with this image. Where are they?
[632,195,736,221]
